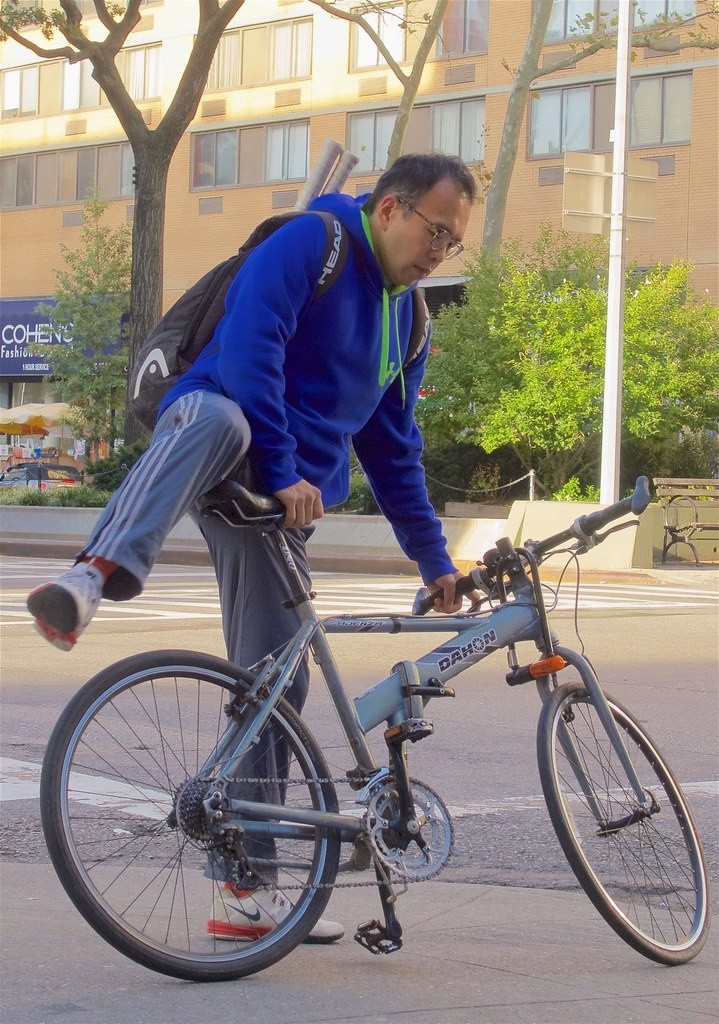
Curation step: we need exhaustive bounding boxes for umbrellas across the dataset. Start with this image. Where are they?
[0,403,88,452]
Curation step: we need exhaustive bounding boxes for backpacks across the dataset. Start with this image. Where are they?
[130,211,431,434]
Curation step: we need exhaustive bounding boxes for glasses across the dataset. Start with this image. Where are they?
[395,196,464,261]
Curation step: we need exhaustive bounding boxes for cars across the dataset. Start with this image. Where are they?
[0,462,82,489]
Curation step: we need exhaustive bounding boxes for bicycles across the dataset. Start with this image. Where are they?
[39,476,710,981]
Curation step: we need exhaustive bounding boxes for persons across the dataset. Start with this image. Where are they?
[24,153,481,941]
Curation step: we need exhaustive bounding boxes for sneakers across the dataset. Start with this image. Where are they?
[207,886,345,944]
[26,558,105,652]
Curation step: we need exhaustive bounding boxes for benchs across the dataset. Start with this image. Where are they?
[652,477,719,567]
